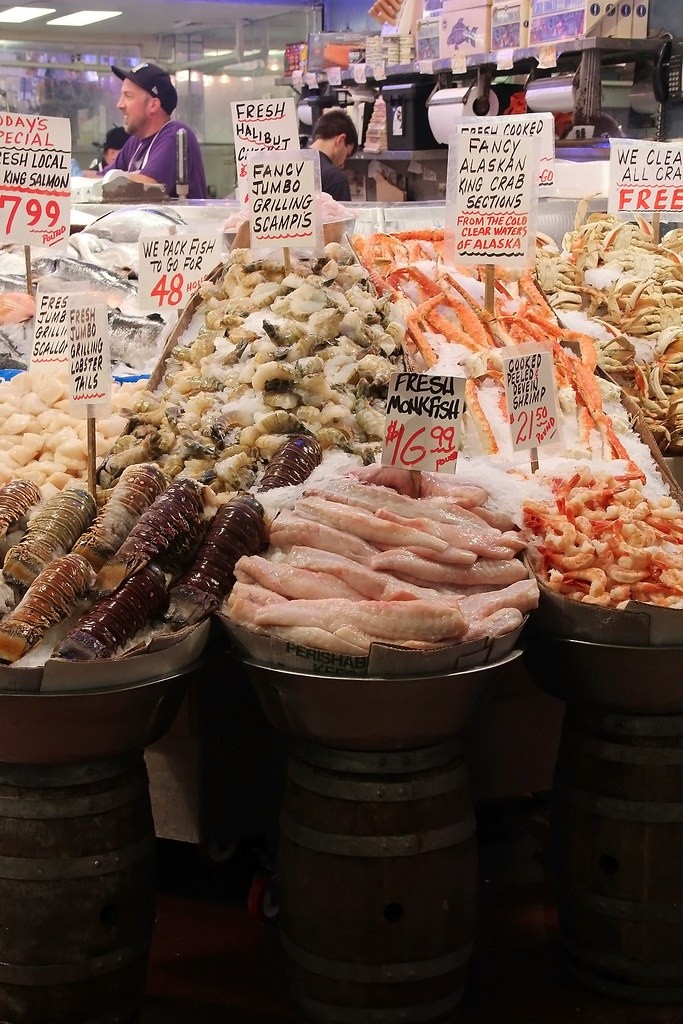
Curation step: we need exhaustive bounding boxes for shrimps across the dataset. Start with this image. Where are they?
[522,465,683,610]
[0,242,407,666]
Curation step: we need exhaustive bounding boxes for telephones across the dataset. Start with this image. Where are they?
[653,37,683,105]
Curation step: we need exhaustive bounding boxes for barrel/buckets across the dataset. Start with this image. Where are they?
[546,704,683,1005]
[0,757,157,1024]
[275,743,480,1023]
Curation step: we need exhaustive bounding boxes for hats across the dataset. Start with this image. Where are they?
[91,126,130,149]
[112,63,179,115]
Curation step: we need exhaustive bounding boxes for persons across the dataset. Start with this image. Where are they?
[69,63,208,200]
[300,109,358,202]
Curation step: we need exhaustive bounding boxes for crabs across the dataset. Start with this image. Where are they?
[350,192,683,485]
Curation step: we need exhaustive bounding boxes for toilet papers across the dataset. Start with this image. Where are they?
[427,85,499,145]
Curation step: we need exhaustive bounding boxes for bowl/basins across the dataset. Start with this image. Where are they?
[227,635,523,749]
[519,627,683,716]
[0,650,209,765]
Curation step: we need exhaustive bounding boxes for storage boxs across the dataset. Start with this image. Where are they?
[381,82,458,151]
